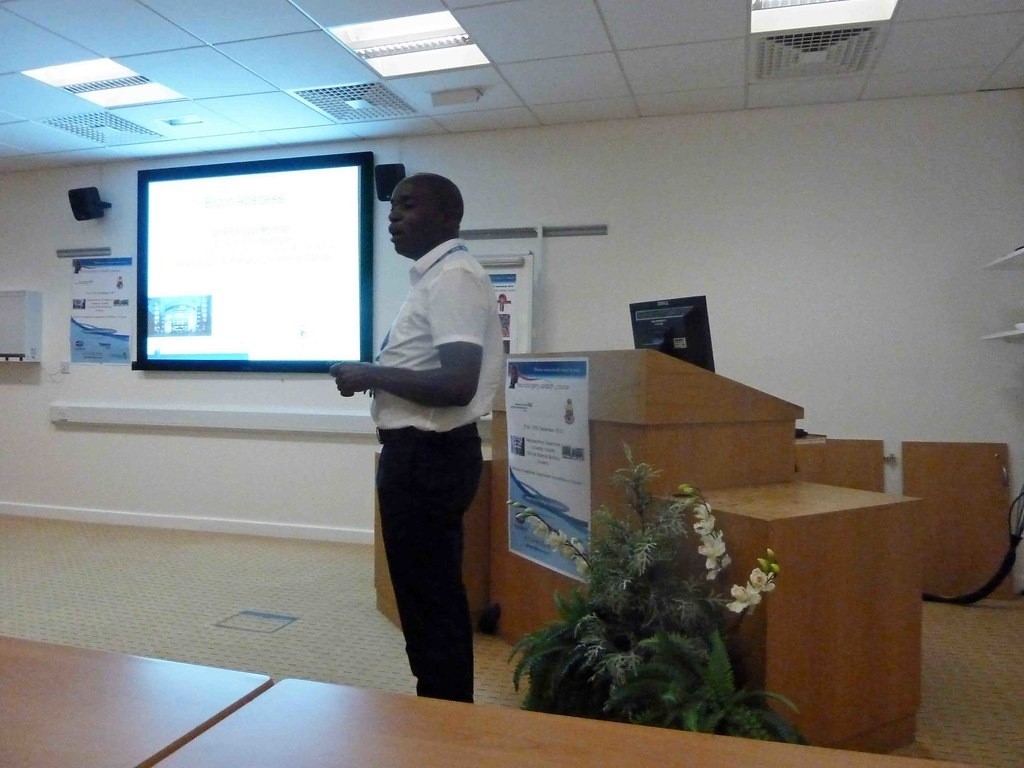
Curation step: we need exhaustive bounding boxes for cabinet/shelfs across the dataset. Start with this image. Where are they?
[642,479,924,751]
[976,247,1024,341]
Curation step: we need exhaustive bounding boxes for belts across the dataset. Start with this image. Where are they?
[376,422,478,443]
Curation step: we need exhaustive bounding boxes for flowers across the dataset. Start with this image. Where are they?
[508,442,808,745]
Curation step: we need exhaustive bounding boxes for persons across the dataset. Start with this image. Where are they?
[330,173,504,704]
[509,365,518,389]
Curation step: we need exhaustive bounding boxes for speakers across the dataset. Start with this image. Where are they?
[375,164,405,201]
[68,187,104,222]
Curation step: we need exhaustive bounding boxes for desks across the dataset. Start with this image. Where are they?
[1,637,972,768]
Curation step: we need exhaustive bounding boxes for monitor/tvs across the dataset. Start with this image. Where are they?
[630,296,716,376]
